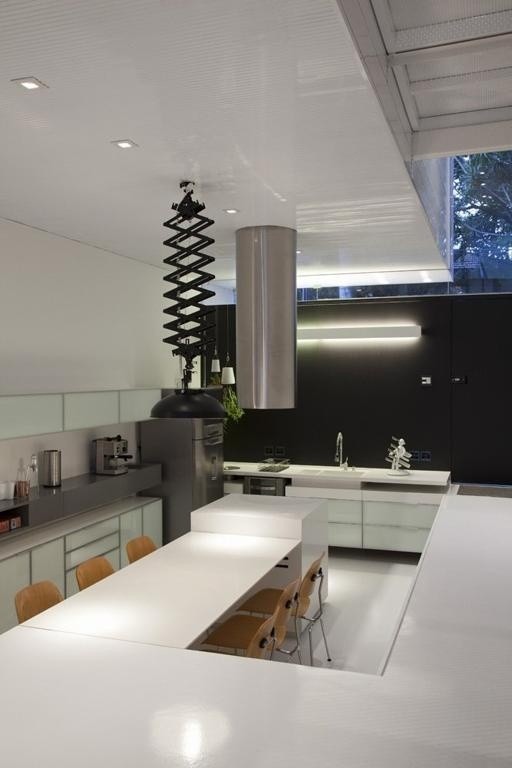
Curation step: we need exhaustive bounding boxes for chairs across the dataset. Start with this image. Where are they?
[15,580,63,624]
[201,578,302,665]
[76,557,115,591]
[126,536,157,564]
[200,606,280,660]
[236,551,332,666]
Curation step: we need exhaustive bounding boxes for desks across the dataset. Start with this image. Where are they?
[20,532,302,648]
[0,494,512,768]
[191,493,330,609]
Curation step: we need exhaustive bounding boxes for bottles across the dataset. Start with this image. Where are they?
[28,454,39,502]
[16,458,26,497]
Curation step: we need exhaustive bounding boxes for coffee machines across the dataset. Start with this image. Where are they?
[96,434,128,476]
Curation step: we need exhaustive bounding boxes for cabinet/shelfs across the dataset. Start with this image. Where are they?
[224,478,451,553]
[0,500,163,634]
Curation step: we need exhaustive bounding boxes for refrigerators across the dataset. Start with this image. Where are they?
[136,418,223,544]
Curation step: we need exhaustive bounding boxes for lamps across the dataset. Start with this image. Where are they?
[150,180,229,420]
[297,326,432,340]
[221,304,235,385]
[211,305,220,373]
